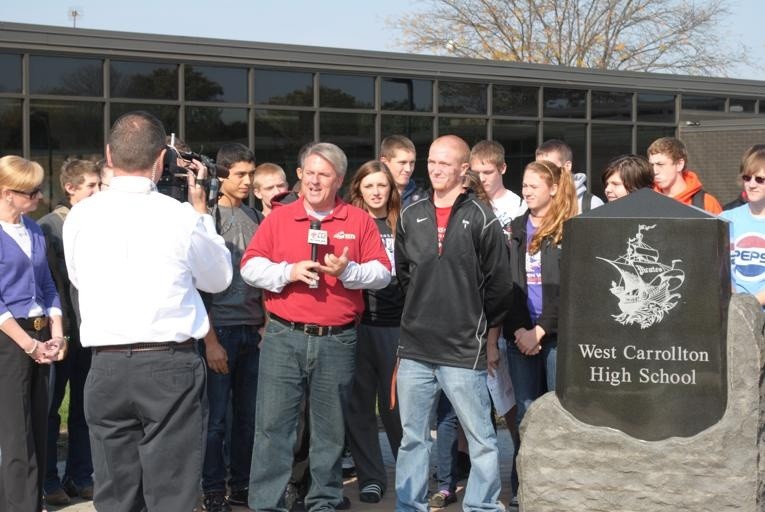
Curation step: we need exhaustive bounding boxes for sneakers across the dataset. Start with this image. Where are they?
[337,497,350,509]
[508,497,520,509]
[43,485,73,507]
[228,486,249,508]
[359,484,384,503]
[62,478,99,501]
[342,467,355,479]
[430,491,458,509]
[204,490,228,512]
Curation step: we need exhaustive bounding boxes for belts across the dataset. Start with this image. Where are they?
[14,316,53,333]
[265,312,361,336]
[88,341,204,352]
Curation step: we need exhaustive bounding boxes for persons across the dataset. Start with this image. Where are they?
[252,163,289,219]
[0,155,63,512]
[198,142,266,512]
[394,134,515,512]
[718,149,765,313]
[36,157,102,505]
[270,142,351,512]
[502,160,578,506]
[239,142,392,512]
[343,160,403,503]
[428,169,508,509]
[471,140,528,438]
[535,139,605,215]
[601,154,655,204]
[380,135,431,211]
[62,111,233,512]
[647,136,723,215]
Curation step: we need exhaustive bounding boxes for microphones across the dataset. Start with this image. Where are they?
[308,220,321,288]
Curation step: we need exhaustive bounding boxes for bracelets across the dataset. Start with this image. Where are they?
[24,338,38,354]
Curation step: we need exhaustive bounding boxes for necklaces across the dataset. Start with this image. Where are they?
[530,213,544,219]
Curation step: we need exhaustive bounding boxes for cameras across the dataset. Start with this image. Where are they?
[156,145,230,209]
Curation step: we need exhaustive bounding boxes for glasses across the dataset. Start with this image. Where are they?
[741,173,765,186]
[7,187,44,202]
